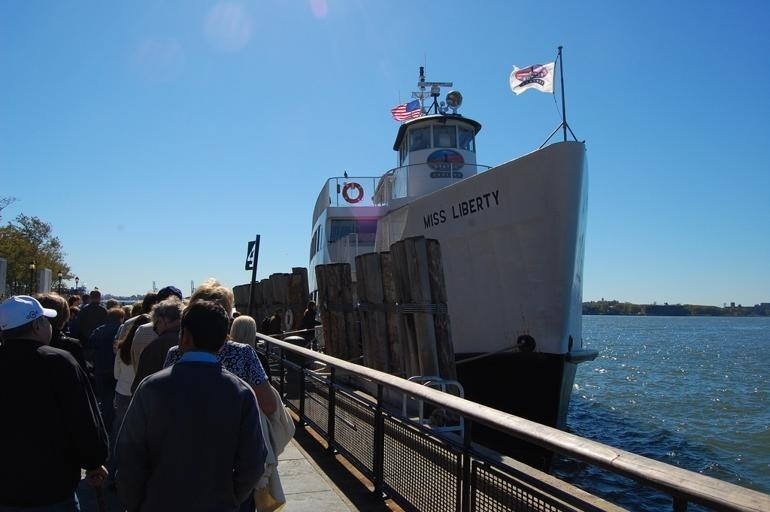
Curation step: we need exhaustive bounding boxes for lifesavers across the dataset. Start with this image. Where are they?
[341,182,364,204]
[284,308,292,330]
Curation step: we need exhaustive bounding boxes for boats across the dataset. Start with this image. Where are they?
[310,44,600,427]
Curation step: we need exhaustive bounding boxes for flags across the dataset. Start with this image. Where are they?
[510,63,555,97]
[392,99,421,122]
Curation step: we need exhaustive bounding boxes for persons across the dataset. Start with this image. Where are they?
[300,301,321,352]
[0,277,287,511]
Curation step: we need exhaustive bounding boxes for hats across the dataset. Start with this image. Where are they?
[157,286,182,301]
[90,291,100,297]
[0,295,58,330]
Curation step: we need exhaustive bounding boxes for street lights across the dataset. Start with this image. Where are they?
[93,286,99,291]
[28,258,36,296]
[57,269,62,296]
[74,276,80,290]
